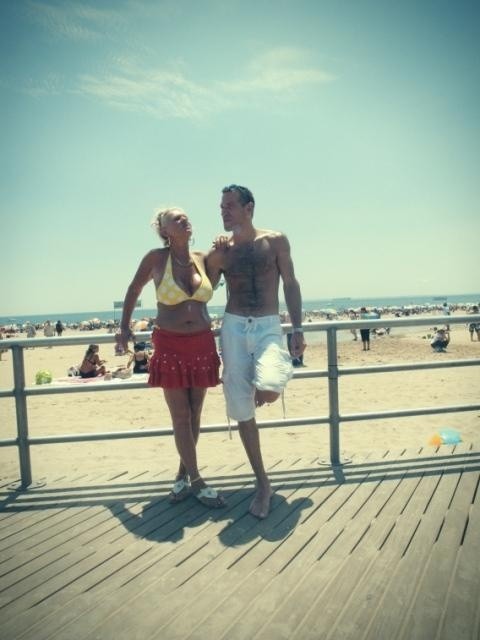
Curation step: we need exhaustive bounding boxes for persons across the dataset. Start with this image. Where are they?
[202,182,308,521]
[431,302,480,352]
[395,308,419,317]
[113,205,231,511]
[1,318,152,379]
[286,306,390,368]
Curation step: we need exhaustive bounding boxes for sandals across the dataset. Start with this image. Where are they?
[191,476,225,509]
[168,472,189,501]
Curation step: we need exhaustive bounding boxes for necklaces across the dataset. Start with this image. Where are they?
[168,246,194,268]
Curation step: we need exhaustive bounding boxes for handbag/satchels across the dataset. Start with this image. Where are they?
[68,367,80,376]
[112,367,131,379]
[36,372,51,384]
